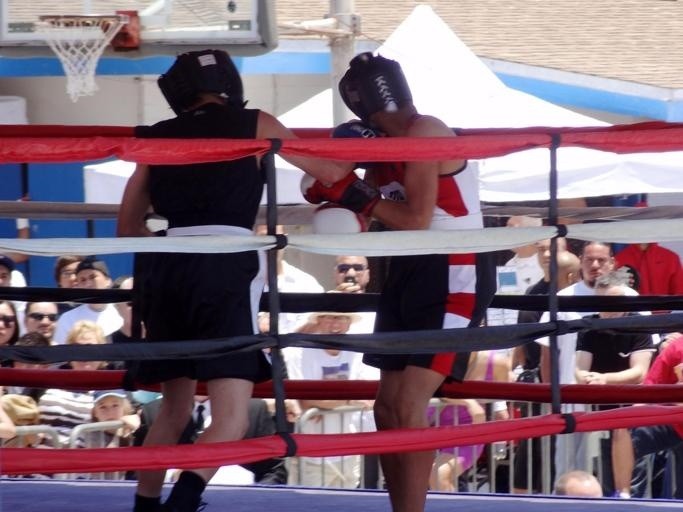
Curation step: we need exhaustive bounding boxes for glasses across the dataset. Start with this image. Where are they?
[337,264,365,273]
[3,316,18,322]
[28,313,59,321]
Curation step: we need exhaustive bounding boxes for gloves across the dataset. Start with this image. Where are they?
[332,120,382,167]
[299,171,382,233]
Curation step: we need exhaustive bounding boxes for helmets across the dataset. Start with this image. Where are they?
[155,48,243,109]
[337,51,415,119]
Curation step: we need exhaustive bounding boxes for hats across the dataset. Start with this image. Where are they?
[1,394,39,426]
[75,256,109,276]
[308,291,363,323]
[93,389,126,402]
[0,254,16,271]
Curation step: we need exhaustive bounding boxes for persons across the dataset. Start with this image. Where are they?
[296,51,500,512]
[115,47,392,511]
